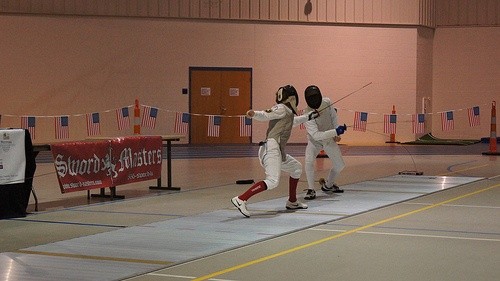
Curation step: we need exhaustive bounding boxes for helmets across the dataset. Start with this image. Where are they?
[304,85,322,109]
[276,85,299,107]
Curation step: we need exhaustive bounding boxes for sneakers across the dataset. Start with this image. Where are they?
[321,183,344,194]
[231,196,250,217]
[303,189,316,200]
[286,200,308,209]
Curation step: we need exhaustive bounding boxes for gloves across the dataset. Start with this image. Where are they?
[308,110,320,121]
[336,125,346,136]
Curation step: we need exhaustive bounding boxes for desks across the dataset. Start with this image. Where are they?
[0,128,36,221]
[30,135,185,212]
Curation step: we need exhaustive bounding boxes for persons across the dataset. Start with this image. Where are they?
[231,85,308,217]
[304,86,348,200]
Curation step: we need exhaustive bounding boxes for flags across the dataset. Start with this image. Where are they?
[353,111,367,132]
[116,107,130,130]
[54,116,70,139]
[21,116,36,141]
[383,114,397,135]
[208,116,221,137]
[239,116,252,137]
[467,106,480,127]
[440,111,455,131]
[174,112,190,135]
[142,107,158,127]
[86,113,101,137]
[411,114,426,134]
[298,109,306,130]
